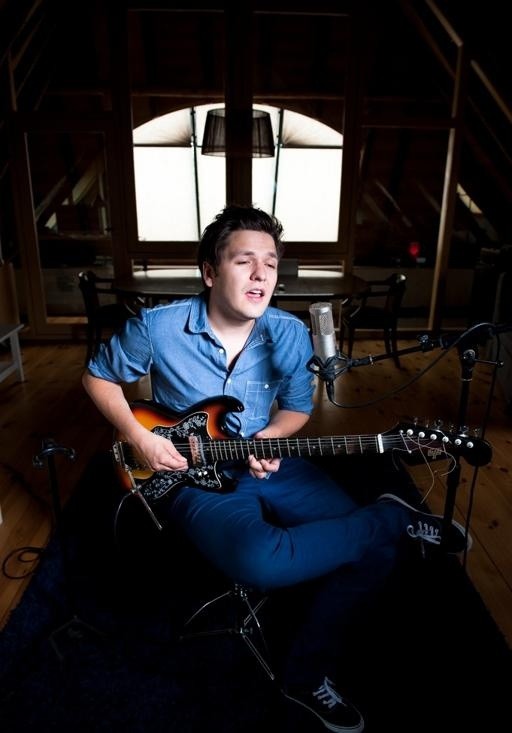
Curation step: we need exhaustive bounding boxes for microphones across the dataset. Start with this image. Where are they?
[308,300,339,400]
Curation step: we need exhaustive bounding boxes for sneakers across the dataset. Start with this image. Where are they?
[276,669,366,733]
[376,490,473,558]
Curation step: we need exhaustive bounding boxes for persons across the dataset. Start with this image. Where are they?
[76,207,473,733]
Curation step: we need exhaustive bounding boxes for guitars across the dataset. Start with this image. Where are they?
[114,394,491,503]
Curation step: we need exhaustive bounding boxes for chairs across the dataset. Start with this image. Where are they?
[78,270,146,369]
[339,272,408,371]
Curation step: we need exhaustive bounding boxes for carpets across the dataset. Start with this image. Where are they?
[1,448,512,733]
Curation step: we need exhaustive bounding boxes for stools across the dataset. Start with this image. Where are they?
[163,505,291,698]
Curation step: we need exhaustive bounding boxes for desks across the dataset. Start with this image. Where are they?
[110,272,370,351]
